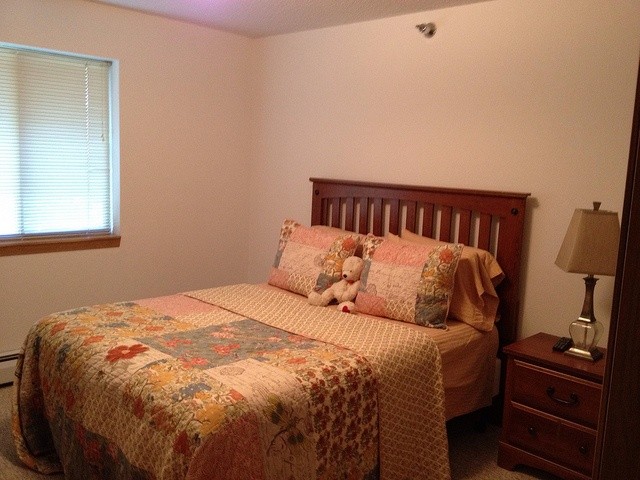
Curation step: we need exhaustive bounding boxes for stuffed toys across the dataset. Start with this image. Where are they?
[305,253,365,313]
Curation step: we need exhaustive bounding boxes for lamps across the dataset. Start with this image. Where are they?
[554,201,620,363]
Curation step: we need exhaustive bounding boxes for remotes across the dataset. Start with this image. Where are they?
[552,337,573,352]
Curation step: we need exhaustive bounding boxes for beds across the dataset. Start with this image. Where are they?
[11,178,533,480]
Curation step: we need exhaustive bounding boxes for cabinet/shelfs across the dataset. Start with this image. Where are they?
[502,332,607,479]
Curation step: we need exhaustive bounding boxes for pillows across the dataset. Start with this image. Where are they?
[270,219,362,304]
[355,234,465,328]
[396,229,492,320]
